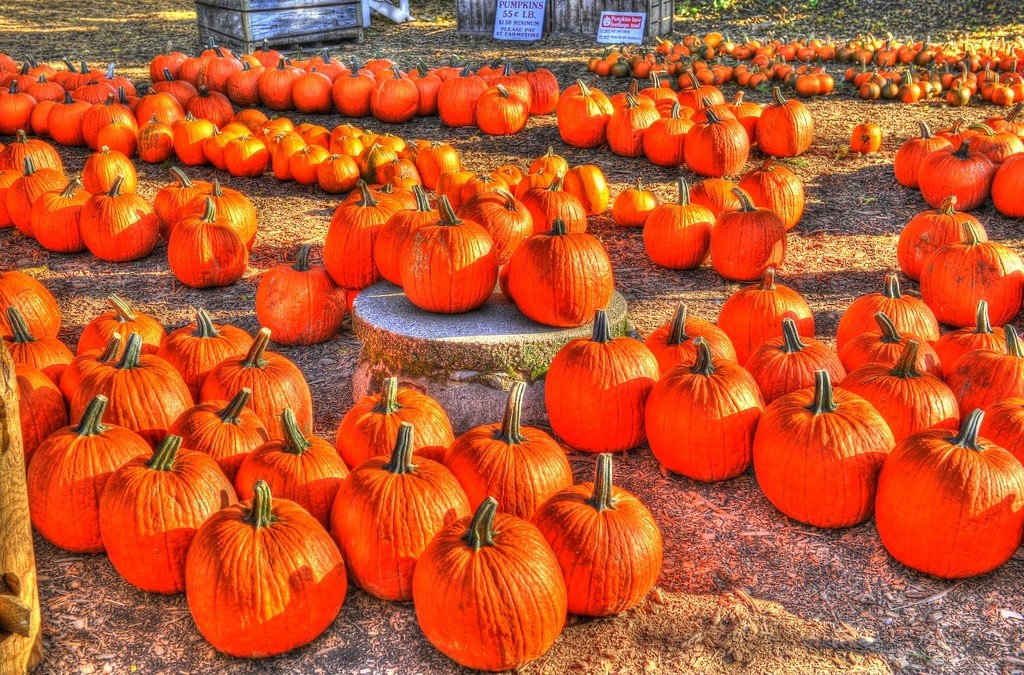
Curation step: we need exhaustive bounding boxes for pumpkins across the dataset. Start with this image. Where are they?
[0,27,1024,675]
